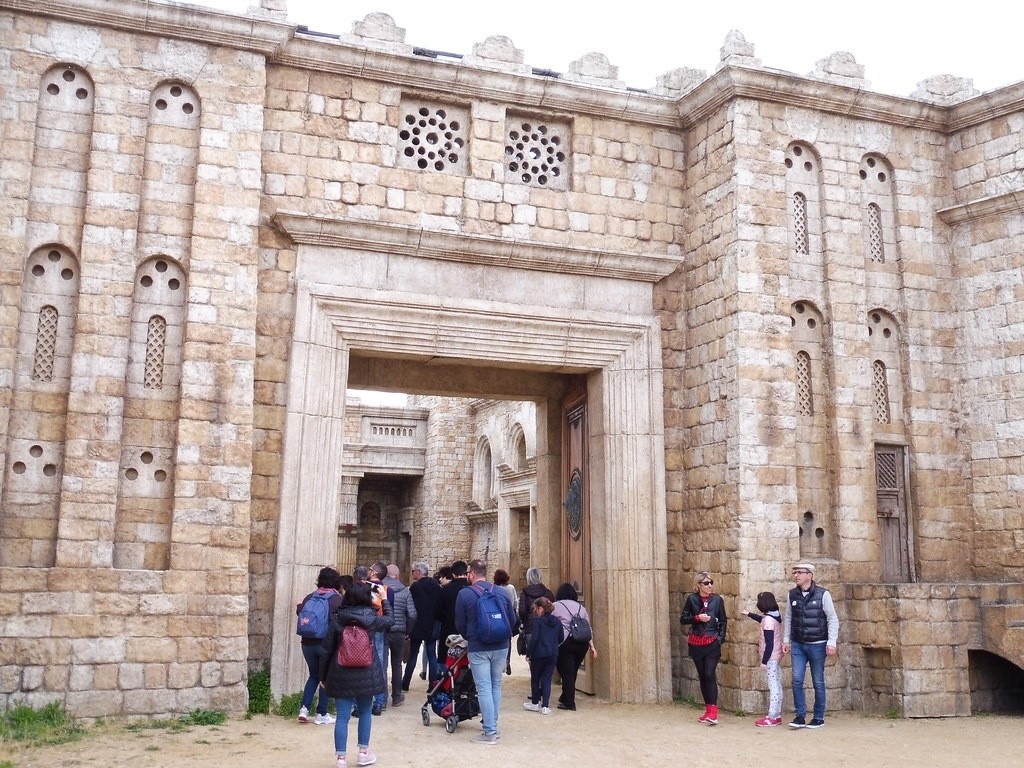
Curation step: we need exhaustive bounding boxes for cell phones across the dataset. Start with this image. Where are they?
[366,581,379,593]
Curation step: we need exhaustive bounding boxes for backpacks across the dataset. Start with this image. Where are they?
[296,591,336,638]
[557,601,591,642]
[466,582,511,644]
[337,620,375,667]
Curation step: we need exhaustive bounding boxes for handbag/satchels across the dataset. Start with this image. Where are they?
[517,631,527,656]
[432,621,442,639]
[403,635,410,663]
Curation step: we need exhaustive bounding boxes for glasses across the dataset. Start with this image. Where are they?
[699,580,714,585]
[464,571,474,576]
[793,572,810,576]
[411,569,421,574]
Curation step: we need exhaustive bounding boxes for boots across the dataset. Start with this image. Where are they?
[698,704,718,724]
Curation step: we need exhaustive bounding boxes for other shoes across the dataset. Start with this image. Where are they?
[523,702,575,714]
[372,706,381,715]
[352,710,360,717]
[391,671,427,706]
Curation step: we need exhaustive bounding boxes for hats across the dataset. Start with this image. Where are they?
[792,564,815,573]
[445,634,468,649]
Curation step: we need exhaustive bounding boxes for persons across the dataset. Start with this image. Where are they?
[401,560,472,694]
[382,564,417,708]
[491,569,519,675]
[518,568,554,700]
[520,597,564,716]
[680,570,727,727]
[457,558,517,745]
[549,582,599,711]
[333,581,393,768]
[296,561,394,725]
[784,561,839,728]
[740,593,783,728]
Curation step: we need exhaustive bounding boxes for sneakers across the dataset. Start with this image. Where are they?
[755,716,781,727]
[788,717,805,728]
[356,750,376,765]
[314,712,337,725]
[805,719,824,728]
[299,708,309,723]
[335,759,348,768]
[470,732,500,745]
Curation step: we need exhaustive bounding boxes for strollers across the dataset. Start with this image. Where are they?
[421,646,483,734]
